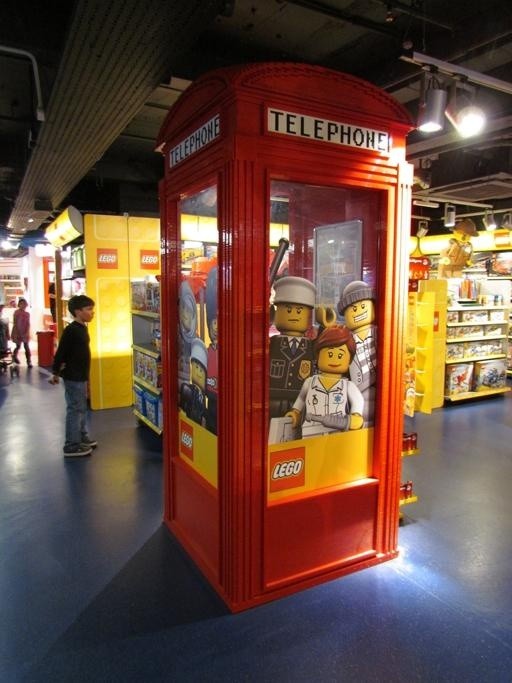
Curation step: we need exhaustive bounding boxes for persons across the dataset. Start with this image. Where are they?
[48,295,98,457]
[11,298,33,368]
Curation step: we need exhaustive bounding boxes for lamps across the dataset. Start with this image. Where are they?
[416,65,488,139]
[411,193,511,239]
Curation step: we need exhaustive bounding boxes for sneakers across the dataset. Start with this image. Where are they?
[81,437,98,447]
[63,442,93,456]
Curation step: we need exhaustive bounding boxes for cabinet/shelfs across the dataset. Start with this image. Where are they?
[130,307,164,436]
[445,305,511,406]
